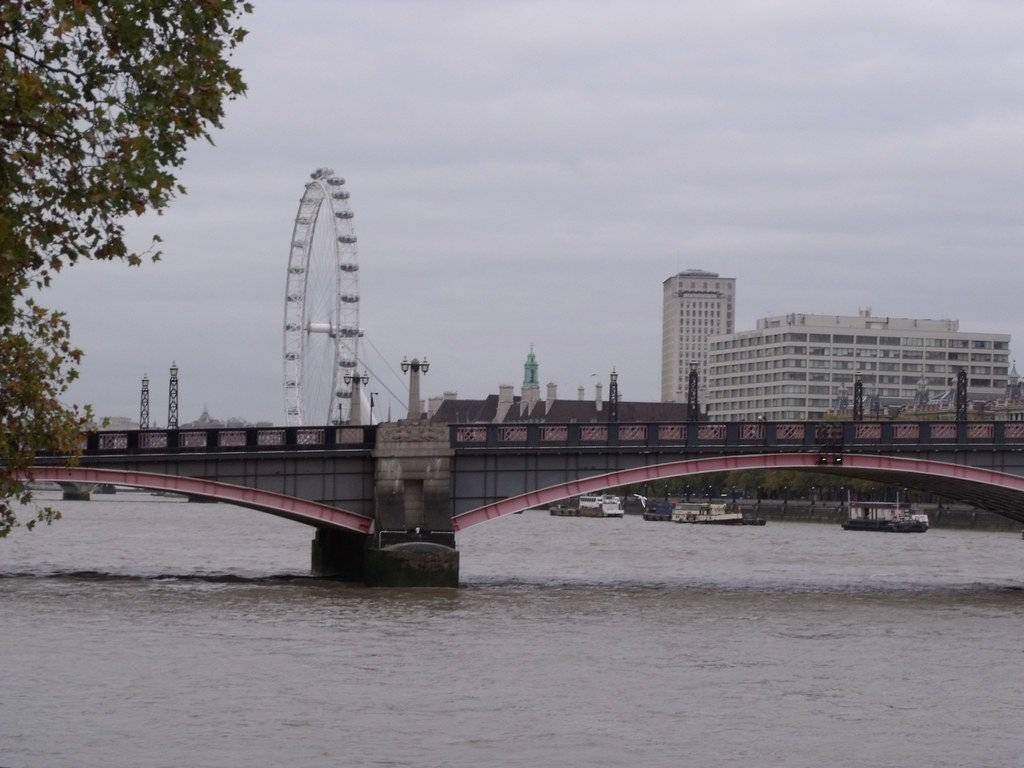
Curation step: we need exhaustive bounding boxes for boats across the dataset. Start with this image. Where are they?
[549,490,624,518]
[640,495,768,526]
[841,488,930,535]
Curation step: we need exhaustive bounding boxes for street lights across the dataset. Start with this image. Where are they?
[343,368,370,425]
[140,371,149,429]
[607,367,619,442]
[400,356,431,425]
[168,361,182,426]
[852,373,864,421]
[687,357,699,446]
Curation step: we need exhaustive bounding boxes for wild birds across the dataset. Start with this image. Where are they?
[633,493,648,509]
